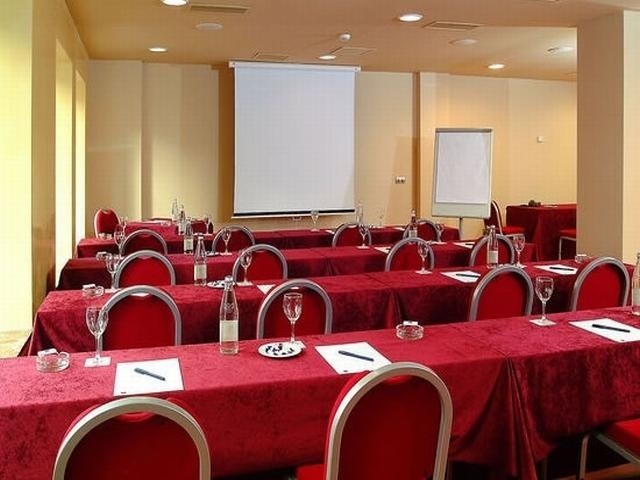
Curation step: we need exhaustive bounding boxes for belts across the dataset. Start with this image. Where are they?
[201,210,252,286]
[108,215,129,292]
[311,209,320,232]
[358,224,369,248]
[282,293,304,345]
[418,240,429,275]
[512,233,526,267]
[408,214,418,245]
[534,276,554,324]
[85,305,108,363]
[436,221,445,242]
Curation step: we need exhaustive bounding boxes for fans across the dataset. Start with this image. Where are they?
[432,127,493,218]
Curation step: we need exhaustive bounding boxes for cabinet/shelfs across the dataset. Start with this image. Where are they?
[219,276,239,355]
[486,225,498,270]
[170,197,209,288]
[631,252,640,316]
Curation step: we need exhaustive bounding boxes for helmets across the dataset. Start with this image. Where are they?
[257,342,302,358]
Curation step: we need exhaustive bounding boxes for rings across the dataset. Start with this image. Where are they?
[549,267,574,271]
[338,351,373,361]
[592,325,630,332]
[386,249,391,250]
[456,274,479,278]
[466,244,474,245]
[134,367,165,381]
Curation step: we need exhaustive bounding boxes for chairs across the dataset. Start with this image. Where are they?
[0,200,639,479]
